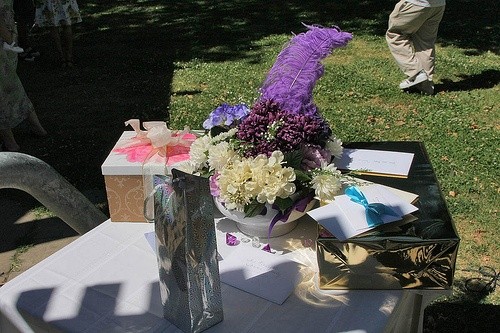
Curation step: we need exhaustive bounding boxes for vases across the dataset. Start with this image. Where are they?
[214,195,315,239]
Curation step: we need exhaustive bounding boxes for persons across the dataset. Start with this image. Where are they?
[385,0,446,94]
[13,0,82,67]
[0,0,47,151]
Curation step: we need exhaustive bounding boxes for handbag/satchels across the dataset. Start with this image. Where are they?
[143,167,225,333]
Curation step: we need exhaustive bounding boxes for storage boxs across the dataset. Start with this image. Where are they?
[101,131,205,223]
[314,141,461,291]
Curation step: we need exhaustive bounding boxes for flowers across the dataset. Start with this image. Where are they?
[189,22,373,236]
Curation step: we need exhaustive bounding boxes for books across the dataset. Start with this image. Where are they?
[330,147,416,179]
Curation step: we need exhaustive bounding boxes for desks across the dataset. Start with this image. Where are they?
[0,188,423,333]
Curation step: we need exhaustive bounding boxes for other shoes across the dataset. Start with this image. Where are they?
[400,69,428,88]
[413,81,436,95]
[17,46,41,64]
